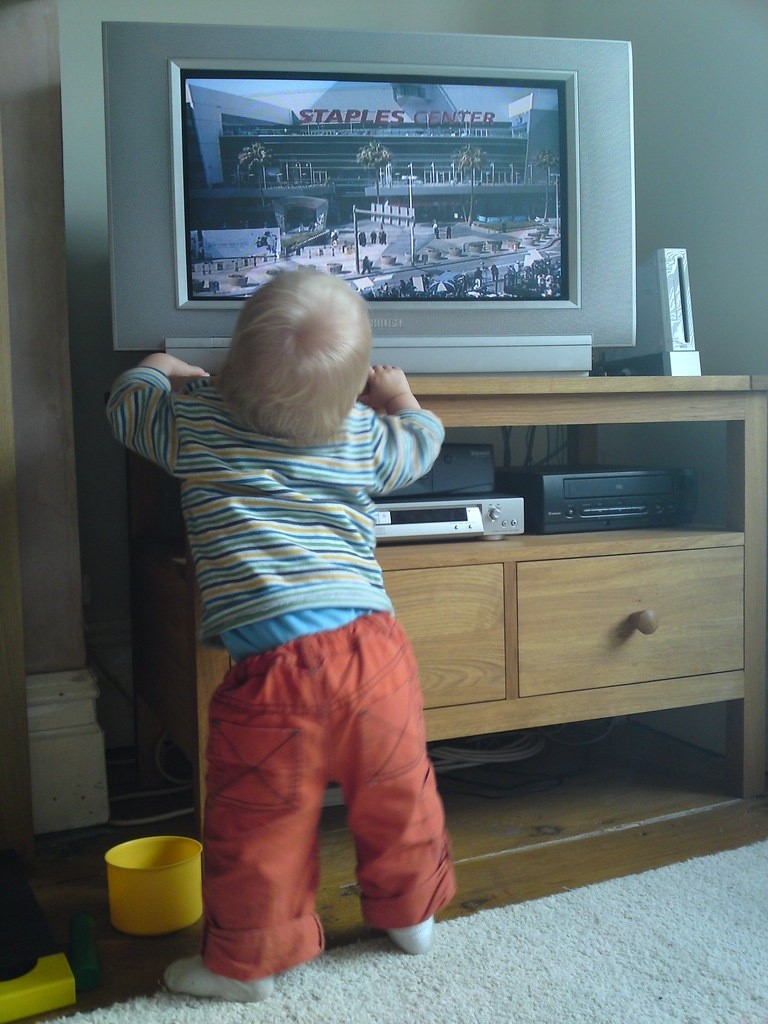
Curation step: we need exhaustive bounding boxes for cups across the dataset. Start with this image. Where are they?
[105,835,202,938]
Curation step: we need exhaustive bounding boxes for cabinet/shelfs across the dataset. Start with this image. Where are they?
[128,372,767,843]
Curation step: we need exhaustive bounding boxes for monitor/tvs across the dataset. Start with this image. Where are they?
[98,19,638,379]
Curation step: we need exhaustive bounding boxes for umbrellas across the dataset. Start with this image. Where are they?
[429,279,454,291]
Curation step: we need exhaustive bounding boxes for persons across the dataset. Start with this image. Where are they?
[435,227,441,239]
[331,230,338,248]
[361,256,371,274]
[364,271,510,298]
[446,226,451,239]
[514,261,521,276]
[473,267,482,287]
[507,258,561,297]
[491,265,499,280]
[358,230,386,247]
[104,270,459,1001]
